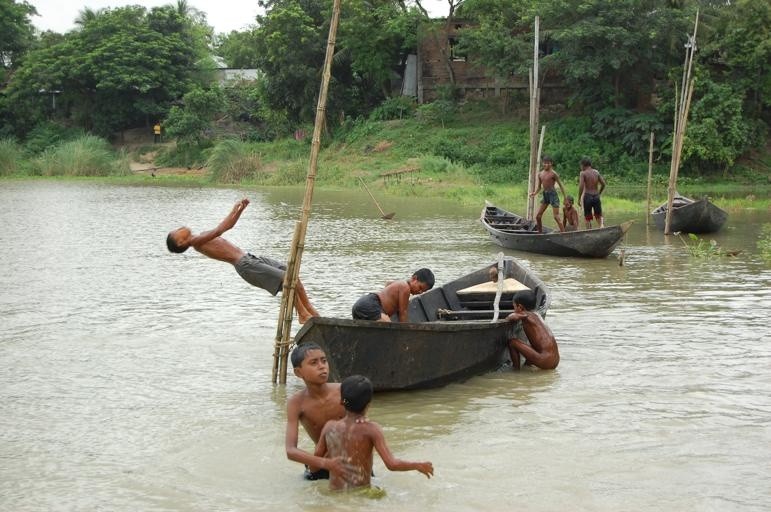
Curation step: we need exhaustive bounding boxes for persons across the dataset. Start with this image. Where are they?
[561,194,580,231]
[351,267,436,323]
[577,157,607,230]
[527,157,571,235]
[285,341,370,481]
[165,198,320,324]
[504,289,561,371]
[307,374,435,493]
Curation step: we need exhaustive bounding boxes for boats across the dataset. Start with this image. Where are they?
[480,192,729,257]
[293,255,552,391]
